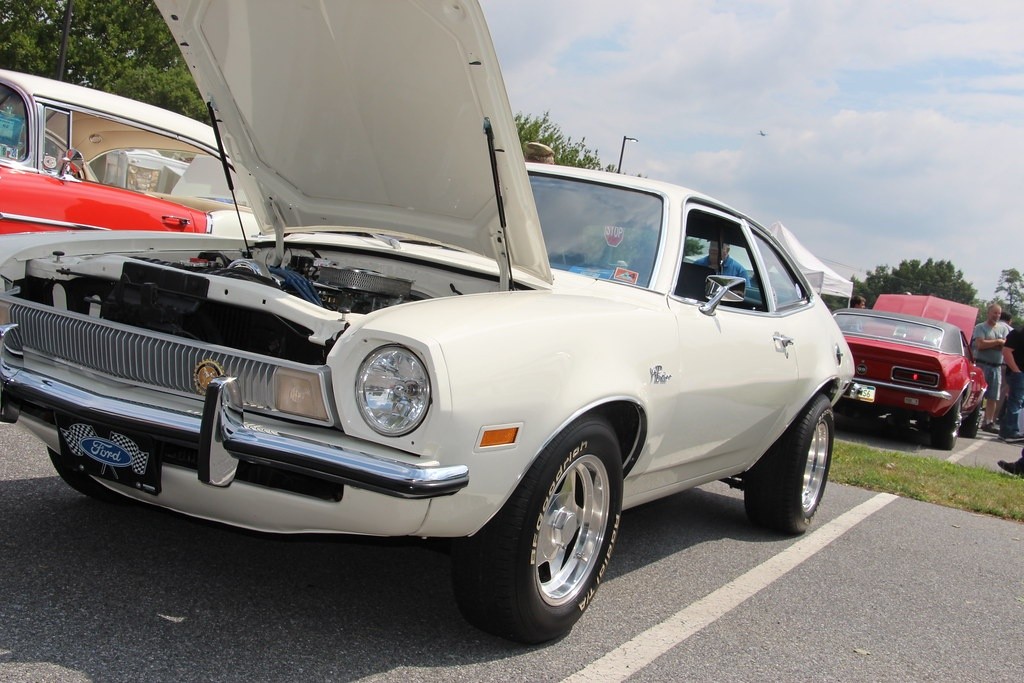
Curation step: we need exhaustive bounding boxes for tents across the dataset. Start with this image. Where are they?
[683,222,853,308]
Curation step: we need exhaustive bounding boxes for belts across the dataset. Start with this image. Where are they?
[976,360,999,368]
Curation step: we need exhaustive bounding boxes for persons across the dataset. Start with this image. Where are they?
[695,239,750,287]
[973,302,1009,433]
[998,448,1024,477]
[999,327,1024,442]
[999,311,1012,325]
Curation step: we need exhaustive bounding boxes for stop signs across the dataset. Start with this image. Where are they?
[603,223,624,248]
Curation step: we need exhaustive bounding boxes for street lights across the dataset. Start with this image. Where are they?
[616,136,639,173]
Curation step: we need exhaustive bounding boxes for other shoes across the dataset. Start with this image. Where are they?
[998,431,1024,442]
[997,457,1024,479]
[982,422,1000,434]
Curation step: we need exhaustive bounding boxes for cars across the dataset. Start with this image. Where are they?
[0,1,859,650]
[0,68,260,237]
[828,295,987,452]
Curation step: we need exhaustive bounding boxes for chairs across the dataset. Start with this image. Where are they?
[674,261,716,302]
